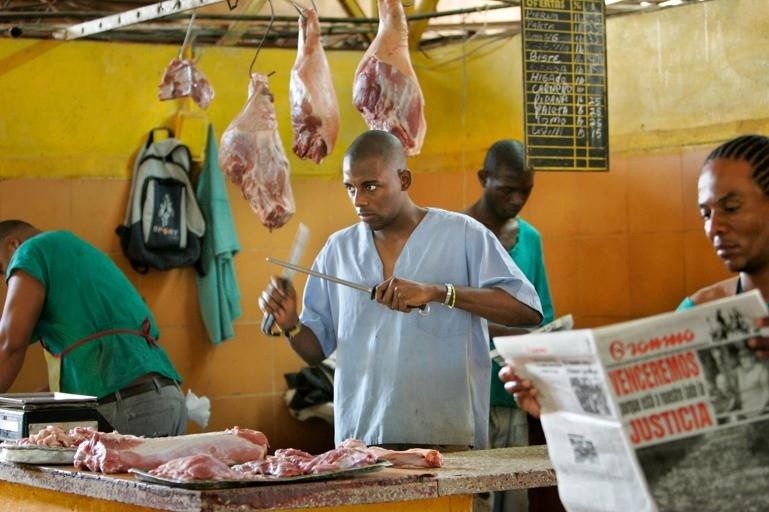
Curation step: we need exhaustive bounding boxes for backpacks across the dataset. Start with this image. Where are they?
[114,126,206,276]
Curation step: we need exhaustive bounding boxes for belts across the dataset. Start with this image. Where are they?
[96,375,181,406]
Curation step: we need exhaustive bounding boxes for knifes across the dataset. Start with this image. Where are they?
[262,222,310,335]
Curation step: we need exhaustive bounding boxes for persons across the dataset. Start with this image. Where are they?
[0,218,191,439]
[255,129,546,512]
[497,132,769,512]
[455,136,558,512]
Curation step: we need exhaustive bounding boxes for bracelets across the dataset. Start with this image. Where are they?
[439,282,452,305]
[268,315,303,340]
[447,282,457,309]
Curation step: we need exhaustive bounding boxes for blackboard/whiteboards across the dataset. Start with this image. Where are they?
[516,0,611,176]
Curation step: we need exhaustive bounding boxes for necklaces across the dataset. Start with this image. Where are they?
[734,275,742,294]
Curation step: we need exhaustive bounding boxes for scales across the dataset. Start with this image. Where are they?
[0,392,114,443]
